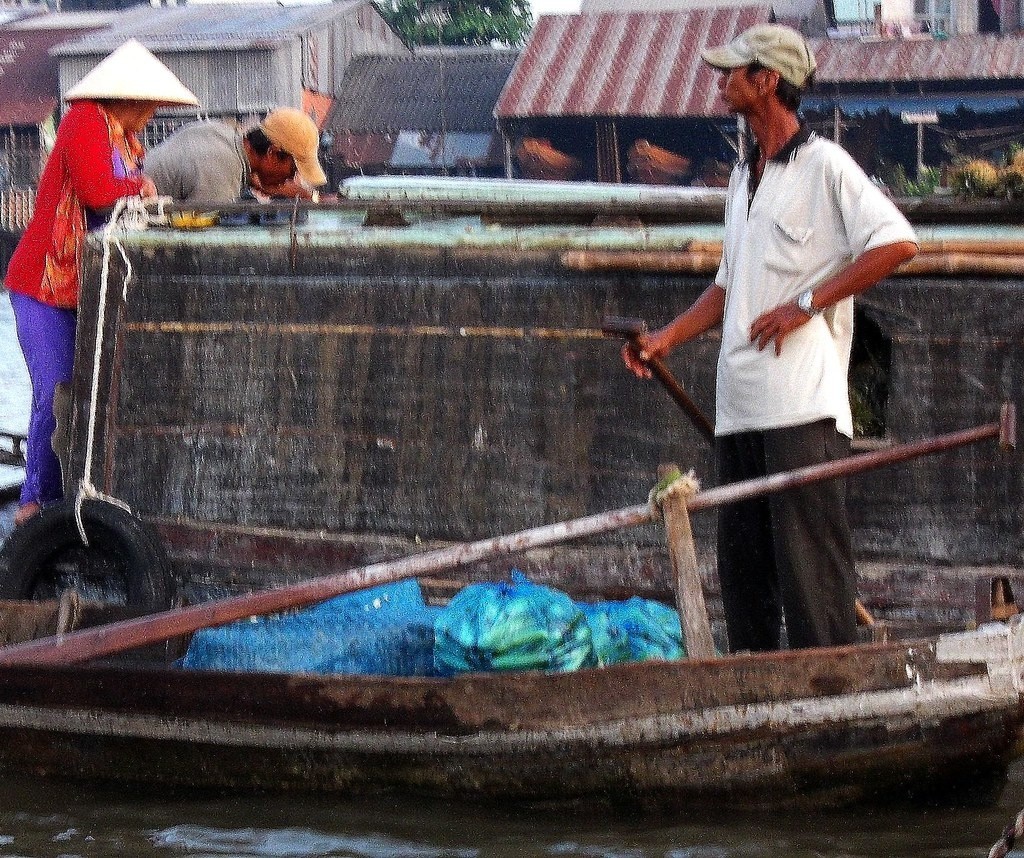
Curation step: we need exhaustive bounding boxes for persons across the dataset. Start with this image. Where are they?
[5,37,202,525]
[621,22,919,654]
[139,103,328,205]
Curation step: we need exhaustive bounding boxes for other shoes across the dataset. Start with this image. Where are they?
[14,501,48,526]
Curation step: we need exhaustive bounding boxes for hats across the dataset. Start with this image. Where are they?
[257,110,327,185]
[701,22,818,91]
[64,37,199,107]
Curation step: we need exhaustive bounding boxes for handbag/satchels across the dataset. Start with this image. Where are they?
[432,570,599,675]
[575,594,688,667]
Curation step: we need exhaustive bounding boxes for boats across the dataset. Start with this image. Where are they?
[0,597,1024,813]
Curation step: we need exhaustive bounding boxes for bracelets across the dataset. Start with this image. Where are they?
[798,290,817,317]
